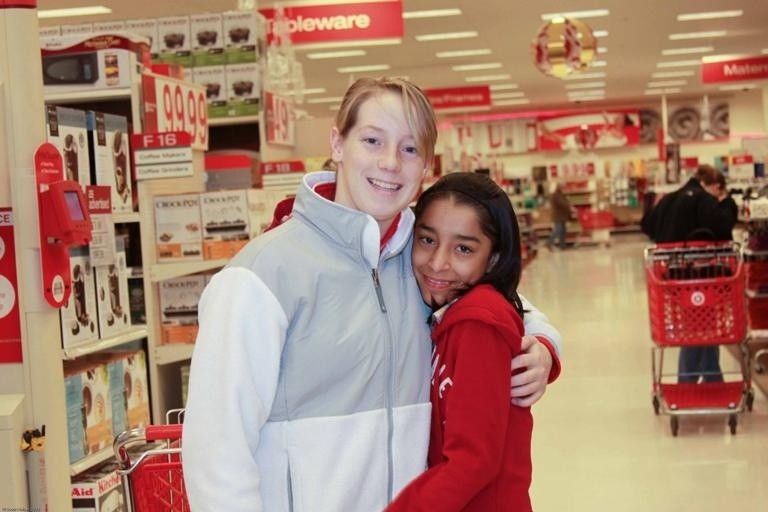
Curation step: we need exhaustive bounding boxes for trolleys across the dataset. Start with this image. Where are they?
[113,408,191,512]
[644,240,753,437]
[570,209,615,248]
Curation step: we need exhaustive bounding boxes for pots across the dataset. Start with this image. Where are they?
[197,31,216,44]
[204,84,219,98]
[164,34,184,48]
[233,80,253,95]
[229,28,249,42]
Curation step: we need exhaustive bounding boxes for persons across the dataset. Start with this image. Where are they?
[640,163,739,383]
[380,170,535,512]
[545,181,577,252]
[181,73,563,511]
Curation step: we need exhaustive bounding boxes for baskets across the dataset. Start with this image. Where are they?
[129,439,191,512]
